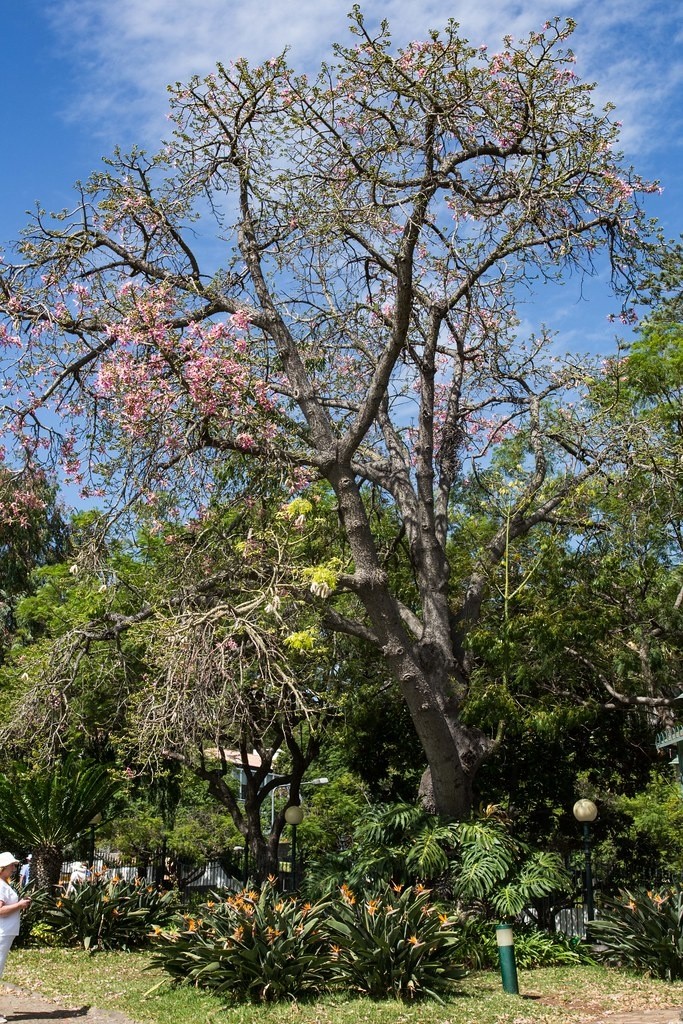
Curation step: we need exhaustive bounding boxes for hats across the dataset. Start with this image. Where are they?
[0,852,19,867]
[26,854,32,859]
[71,862,86,871]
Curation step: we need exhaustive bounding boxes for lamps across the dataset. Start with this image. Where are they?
[495,924,514,946]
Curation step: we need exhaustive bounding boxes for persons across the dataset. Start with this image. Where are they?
[20,853,33,892]
[0,852,32,1024]
[66,861,91,896]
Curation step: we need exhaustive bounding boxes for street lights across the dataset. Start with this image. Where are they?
[573,799,599,923]
[281,805,304,896]
[88,809,102,871]
[270,778,329,838]
